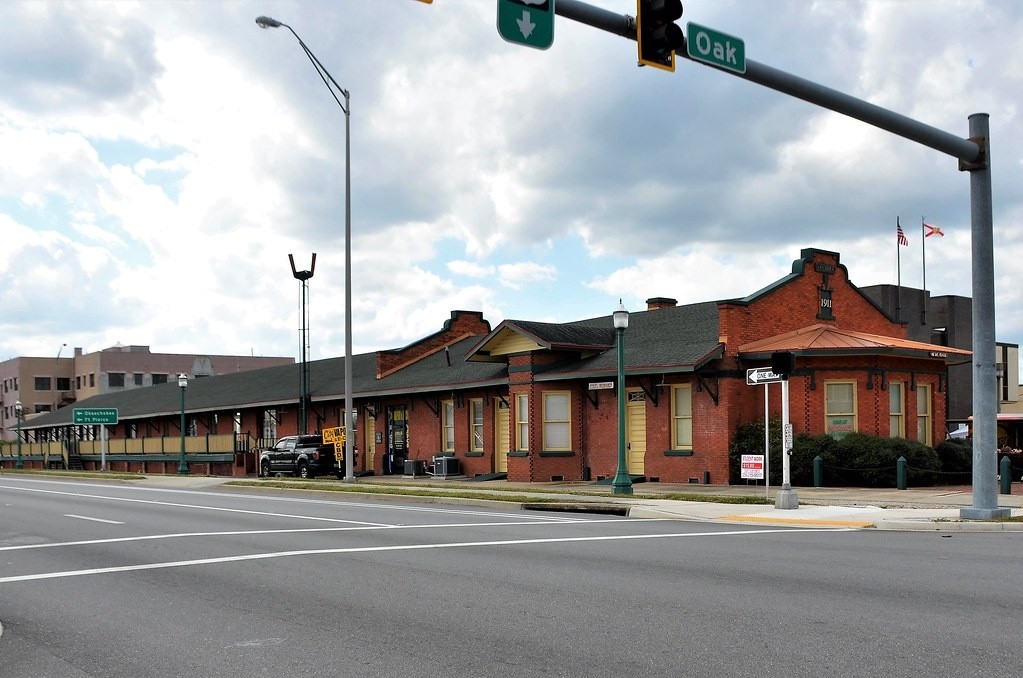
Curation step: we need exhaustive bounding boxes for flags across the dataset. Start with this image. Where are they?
[898,226,908,246]
[924,224,944,238]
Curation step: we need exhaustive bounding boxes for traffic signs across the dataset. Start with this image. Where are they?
[747,367,782,385]
[72,407,118,424]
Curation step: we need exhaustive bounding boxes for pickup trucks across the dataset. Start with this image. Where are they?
[260,434,359,480]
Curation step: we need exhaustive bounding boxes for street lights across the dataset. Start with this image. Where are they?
[15,401,23,469]
[612,298,634,495]
[257,15,356,482]
[177,372,189,476]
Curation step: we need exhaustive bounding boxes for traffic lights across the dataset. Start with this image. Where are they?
[637,1,683,71]
[771,351,793,373]
